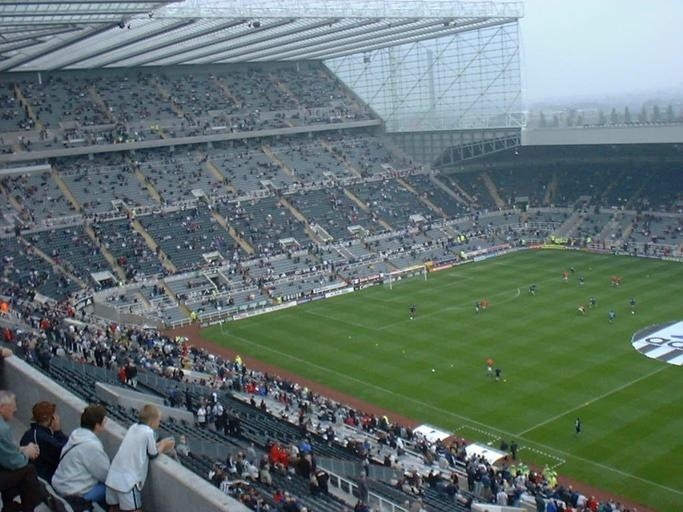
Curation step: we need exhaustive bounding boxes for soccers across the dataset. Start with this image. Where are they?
[432,369,435,372]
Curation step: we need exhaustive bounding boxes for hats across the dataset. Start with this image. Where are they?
[30,402,55,419]
